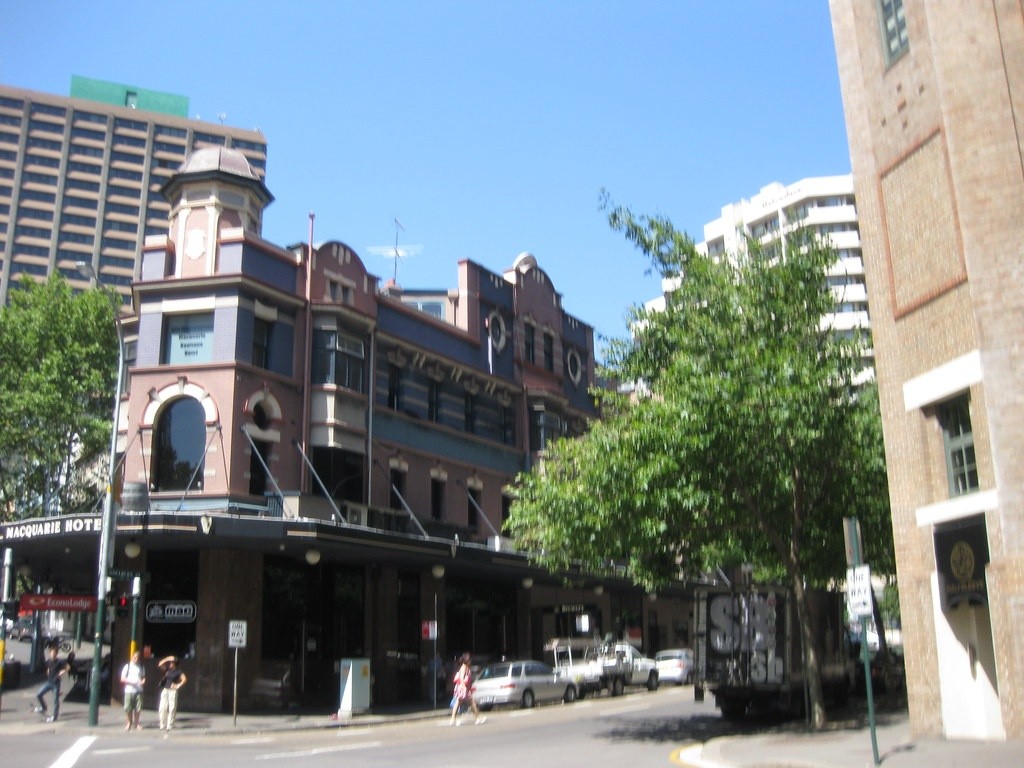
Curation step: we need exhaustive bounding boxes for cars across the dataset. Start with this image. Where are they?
[472,659,580,710]
[10,617,33,643]
[655,645,695,686]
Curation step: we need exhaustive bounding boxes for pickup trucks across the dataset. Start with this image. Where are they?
[558,640,662,695]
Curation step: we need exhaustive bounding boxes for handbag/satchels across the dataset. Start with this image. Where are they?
[159,668,168,687]
[453,666,469,685]
[449,696,464,713]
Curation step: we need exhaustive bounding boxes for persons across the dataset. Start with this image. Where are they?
[158,656,187,738]
[448,653,486,726]
[35,644,70,723]
[429,653,446,693]
[121,650,146,731]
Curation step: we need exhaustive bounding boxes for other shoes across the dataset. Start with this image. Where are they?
[475,716,486,724]
[126,723,132,731]
[450,719,461,726]
[47,716,57,722]
[34,706,48,713]
[135,723,142,732]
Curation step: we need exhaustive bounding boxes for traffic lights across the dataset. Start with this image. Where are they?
[112,593,130,610]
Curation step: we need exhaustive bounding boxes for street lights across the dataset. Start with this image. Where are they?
[74,259,127,730]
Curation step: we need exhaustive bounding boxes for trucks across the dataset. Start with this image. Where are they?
[694,583,857,724]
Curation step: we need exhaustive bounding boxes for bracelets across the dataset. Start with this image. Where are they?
[176,683,180,690]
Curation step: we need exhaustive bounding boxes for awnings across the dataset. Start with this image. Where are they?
[1,510,734,596]
